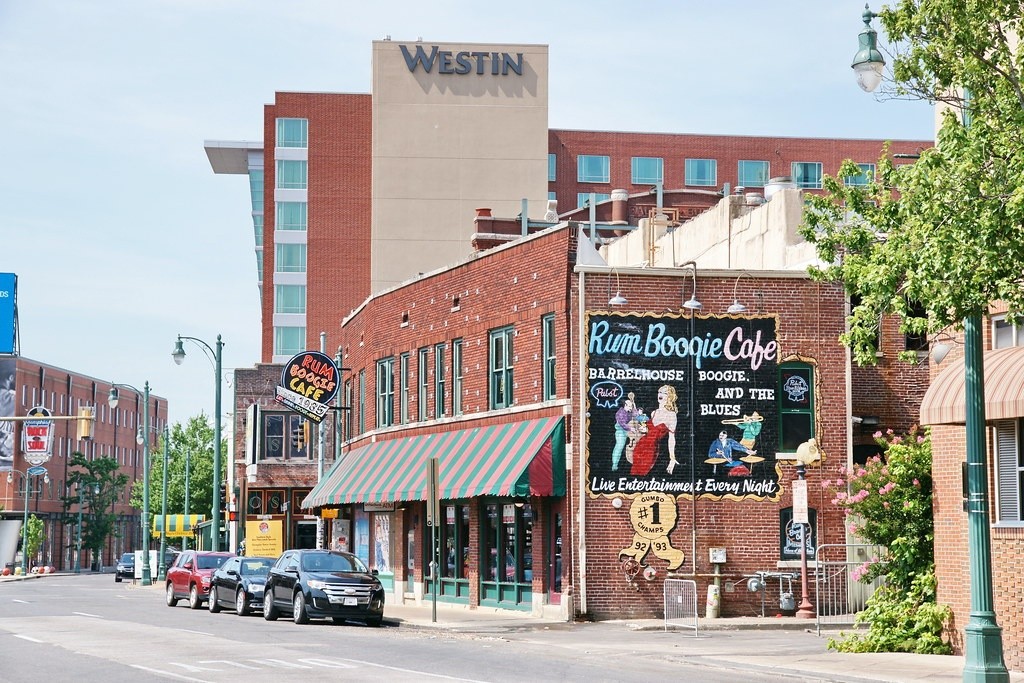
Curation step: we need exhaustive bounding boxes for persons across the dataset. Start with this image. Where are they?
[0,360,16,456]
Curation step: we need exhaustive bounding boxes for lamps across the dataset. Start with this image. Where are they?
[608,267,629,306]
[931,332,965,366]
[682,269,702,309]
[726,272,763,314]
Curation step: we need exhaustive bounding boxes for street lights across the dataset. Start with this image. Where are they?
[848,2,1012,683]
[107,380,152,586]
[166,448,189,551]
[6,468,49,577]
[136,421,167,582]
[171,332,225,553]
[74,481,100,573]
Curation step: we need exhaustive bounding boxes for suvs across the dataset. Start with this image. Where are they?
[263,548,385,627]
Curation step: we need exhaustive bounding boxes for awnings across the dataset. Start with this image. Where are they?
[301,415,567,509]
[920,347,1024,426]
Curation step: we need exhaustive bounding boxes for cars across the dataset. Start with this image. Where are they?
[166,549,237,610]
[208,556,278,617]
[114,552,134,582]
[445,546,562,588]
[157,546,180,576]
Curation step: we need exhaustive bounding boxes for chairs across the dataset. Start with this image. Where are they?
[243,564,254,574]
[305,556,316,568]
